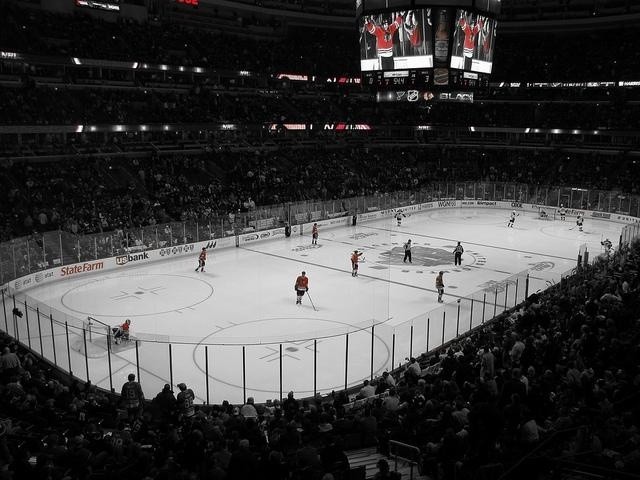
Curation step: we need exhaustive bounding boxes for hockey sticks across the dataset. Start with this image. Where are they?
[307,291,319,311]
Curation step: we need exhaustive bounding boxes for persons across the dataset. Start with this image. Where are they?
[508,209,520,227]
[393,209,407,225]
[112,317,133,345]
[360,92,639,218]
[576,215,584,231]
[403,239,413,263]
[453,242,464,266]
[311,223,319,245]
[196,247,207,272]
[191,26,360,244]
[1,329,639,480]
[583,237,640,328]
[480,269,583,329]
[0,10,191,286]
[351,250,363,277]
[293,271,310,306]
[434,270,447,304]
[558,205,567,221]
[363,11,492,73]
